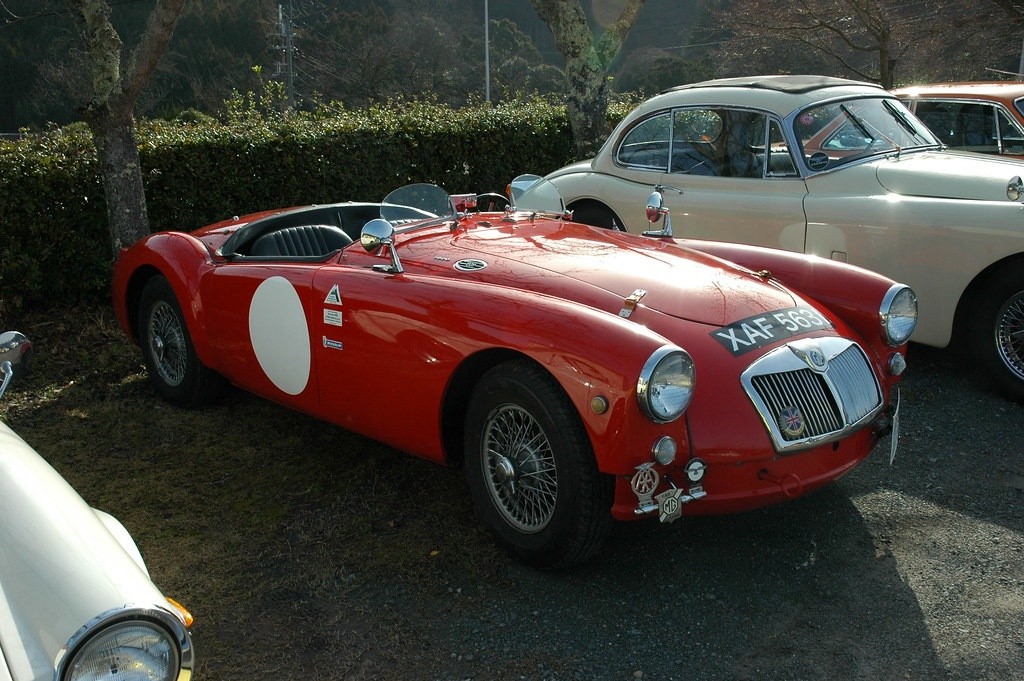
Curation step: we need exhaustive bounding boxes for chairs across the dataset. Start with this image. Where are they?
[253,224,353,263]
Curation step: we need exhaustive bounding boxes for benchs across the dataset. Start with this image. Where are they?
[671,152,796,178]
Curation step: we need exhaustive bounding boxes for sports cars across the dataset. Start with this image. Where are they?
[112,174,918,557]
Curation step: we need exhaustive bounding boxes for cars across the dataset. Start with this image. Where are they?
[516,75,1024,403]
[755,79,1024,160]
